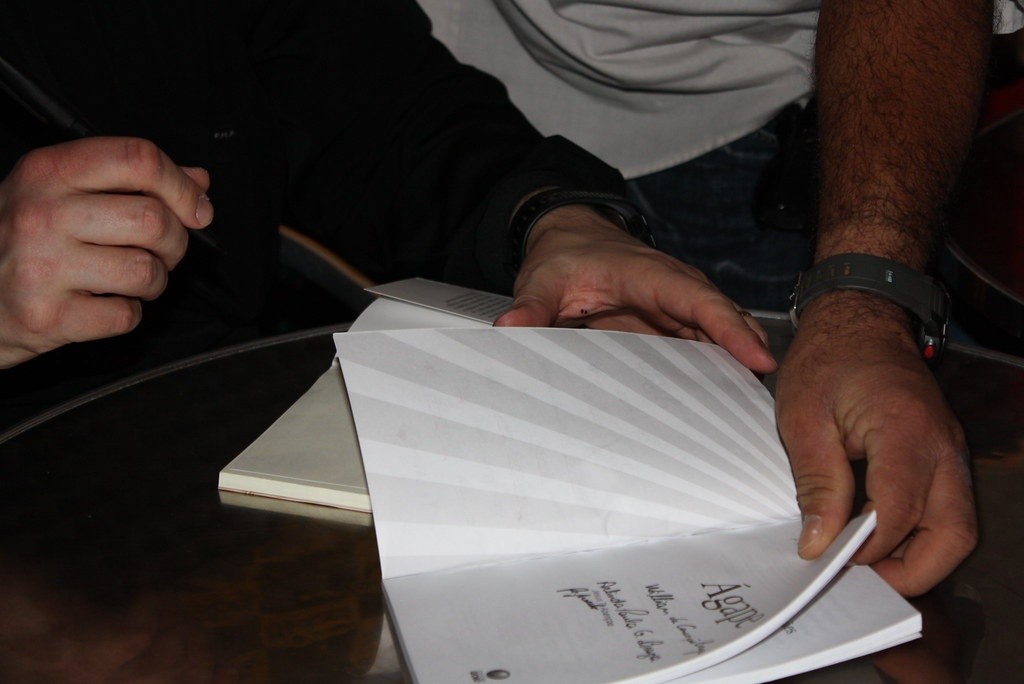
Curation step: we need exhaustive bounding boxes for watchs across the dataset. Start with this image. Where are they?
[790,251,951,372]
[503,190,655,282]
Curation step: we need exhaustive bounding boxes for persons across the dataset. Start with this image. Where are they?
[419,0,1024,597]
[0,0,780,423]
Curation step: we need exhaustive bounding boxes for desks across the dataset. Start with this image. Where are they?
[0,302,1024,683]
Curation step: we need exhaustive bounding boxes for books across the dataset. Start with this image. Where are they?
[213,276,521,529]
[323,323,920,684]
